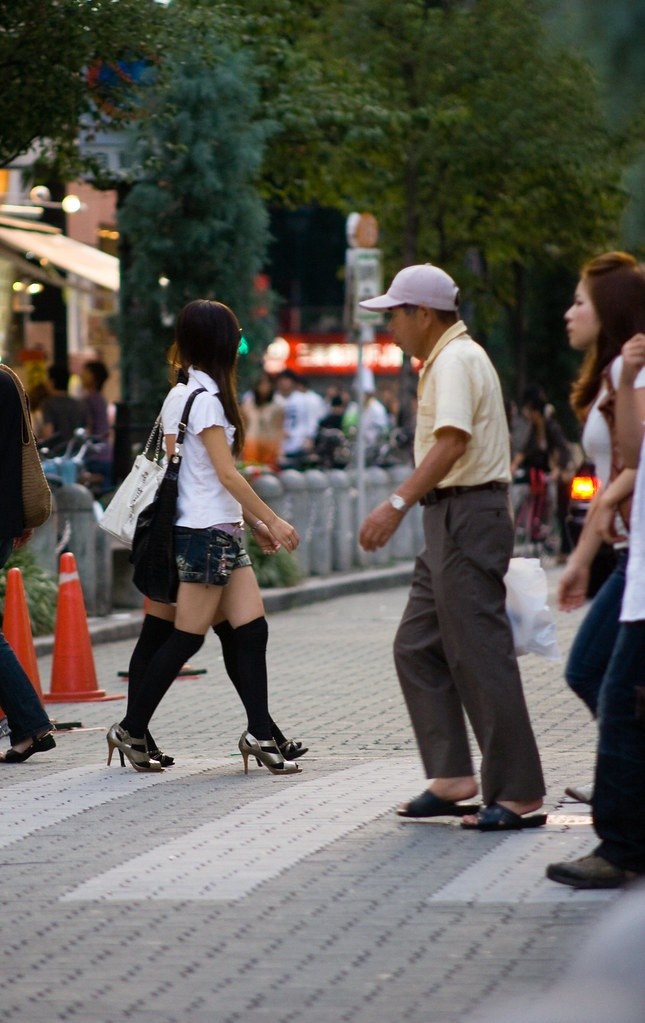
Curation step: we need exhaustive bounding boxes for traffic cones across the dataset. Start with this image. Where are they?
[117,596,199,680]
[0,569,42,715]
[41,553,125,704]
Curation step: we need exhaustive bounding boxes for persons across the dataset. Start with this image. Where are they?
[533,246,645,896]
[0,365,57,763]
[351,260,553,833]
[89,300,309,778]
[22,327,600,564]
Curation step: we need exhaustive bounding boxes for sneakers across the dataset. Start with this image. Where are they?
[546,852,639,889]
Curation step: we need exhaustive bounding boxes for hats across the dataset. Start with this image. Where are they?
[359,263,461,311]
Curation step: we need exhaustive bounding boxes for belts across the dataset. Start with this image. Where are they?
[419,481,508,507]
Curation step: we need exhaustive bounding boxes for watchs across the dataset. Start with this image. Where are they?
[387,493,409,514]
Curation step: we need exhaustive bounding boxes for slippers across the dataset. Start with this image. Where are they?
[460,802,547,831]
[396,789,483,817]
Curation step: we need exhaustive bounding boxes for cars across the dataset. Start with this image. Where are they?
[567,461,601,535]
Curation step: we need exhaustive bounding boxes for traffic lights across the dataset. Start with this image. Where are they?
[238,338,250,354]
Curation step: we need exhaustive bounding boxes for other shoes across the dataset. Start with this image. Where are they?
[0,734,56,763]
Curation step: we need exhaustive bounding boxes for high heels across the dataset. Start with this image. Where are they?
[106,723,161,772]
[255,739,308,767]
[118,729,175,767]
[239,731,302,775]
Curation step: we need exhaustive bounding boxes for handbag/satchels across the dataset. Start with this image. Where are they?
[129,387,207,603]
[0,363,52,529]
[97,412,167,545]
[504,557,560,659]
